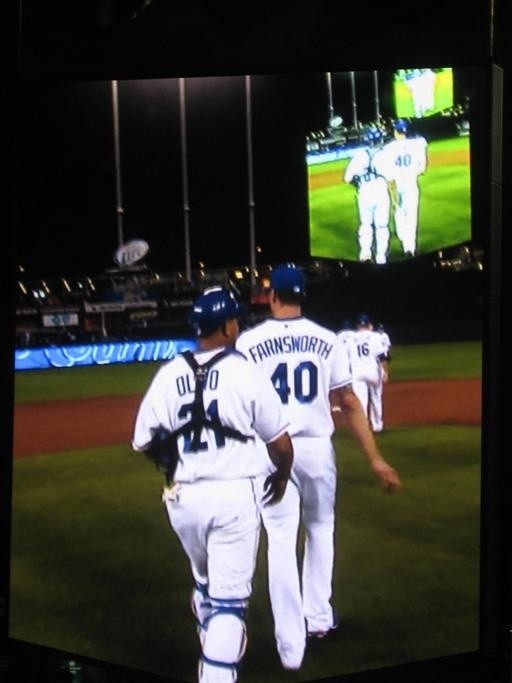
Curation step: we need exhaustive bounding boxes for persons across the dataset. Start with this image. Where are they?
[369,118,430,257]
[403,68,423,119]
[404,132,430,176]
[418,68,436,113]
[332,313,392,435]
[233,264,403,673]
[342,123,392,265]
[129,281,297,683]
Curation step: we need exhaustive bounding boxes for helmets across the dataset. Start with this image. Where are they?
[188,284,243,322]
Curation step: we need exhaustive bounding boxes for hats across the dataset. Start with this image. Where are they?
[365,117,413,140]
[269,261,309,294]
